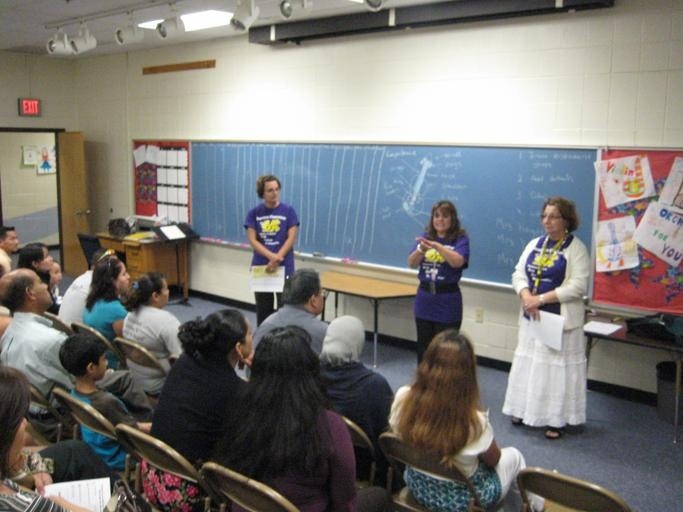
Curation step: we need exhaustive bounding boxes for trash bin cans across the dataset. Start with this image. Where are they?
[655,361,683,425]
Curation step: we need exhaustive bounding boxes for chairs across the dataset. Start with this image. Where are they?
[328,410,389,512]
[1,305,302,512]
[76,233,105,271]
[515,467,637,511]
[377,430,522,512]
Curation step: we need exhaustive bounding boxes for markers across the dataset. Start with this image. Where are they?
[239,243,249,248]
[313,252,324,257]
[342,258,351,264]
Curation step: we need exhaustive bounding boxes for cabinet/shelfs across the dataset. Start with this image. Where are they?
[94,231,192,306]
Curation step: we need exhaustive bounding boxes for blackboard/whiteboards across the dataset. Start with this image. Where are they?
[188,139,603,301]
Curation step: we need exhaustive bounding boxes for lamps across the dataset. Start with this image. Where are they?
[39,0,386,59]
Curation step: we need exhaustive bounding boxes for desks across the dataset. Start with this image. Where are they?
[310,269,420,369]
[572,311,683,444]
